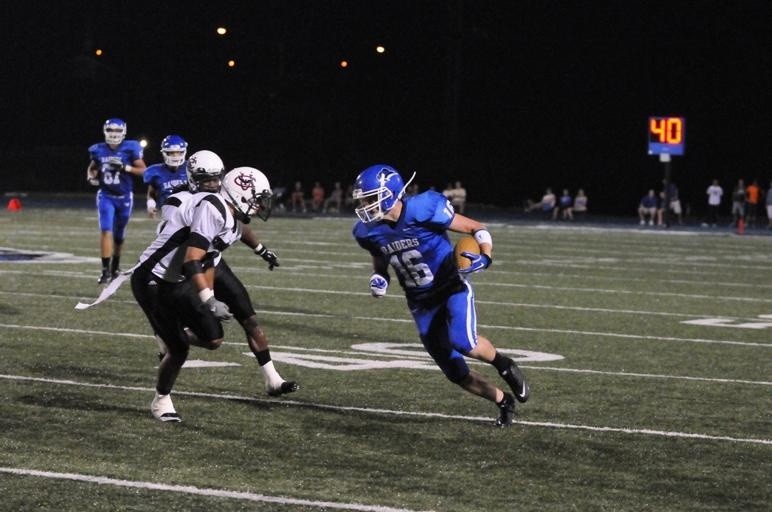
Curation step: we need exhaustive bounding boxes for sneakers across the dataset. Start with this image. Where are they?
[493,391,515,429]
[266,377,300,398]
[97,267,121,284]
[148,393,184,425]
[498,358,531,404]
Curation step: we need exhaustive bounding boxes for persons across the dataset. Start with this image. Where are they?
[638,179,682,227]
[85,119,148,285]
[703,178,760,230]
[350,164,533,432]
[130,166,301,425]
[541,187,587,220]
[143,134,193,237]
[272,179,358,216]
[443,182,466,213]
[154,149,299,398]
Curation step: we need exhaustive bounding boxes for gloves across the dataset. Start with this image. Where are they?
[370,273,390,297]
[108,158,131,172]
[457,252,493,280]
[197,288,235,321]
[253,242,281,271]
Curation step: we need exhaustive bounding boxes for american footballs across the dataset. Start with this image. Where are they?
[454,236,481,270]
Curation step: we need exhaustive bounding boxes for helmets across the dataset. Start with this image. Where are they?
[351,163,407,225]
[159,133,275,223]
[101,117,128,145]
